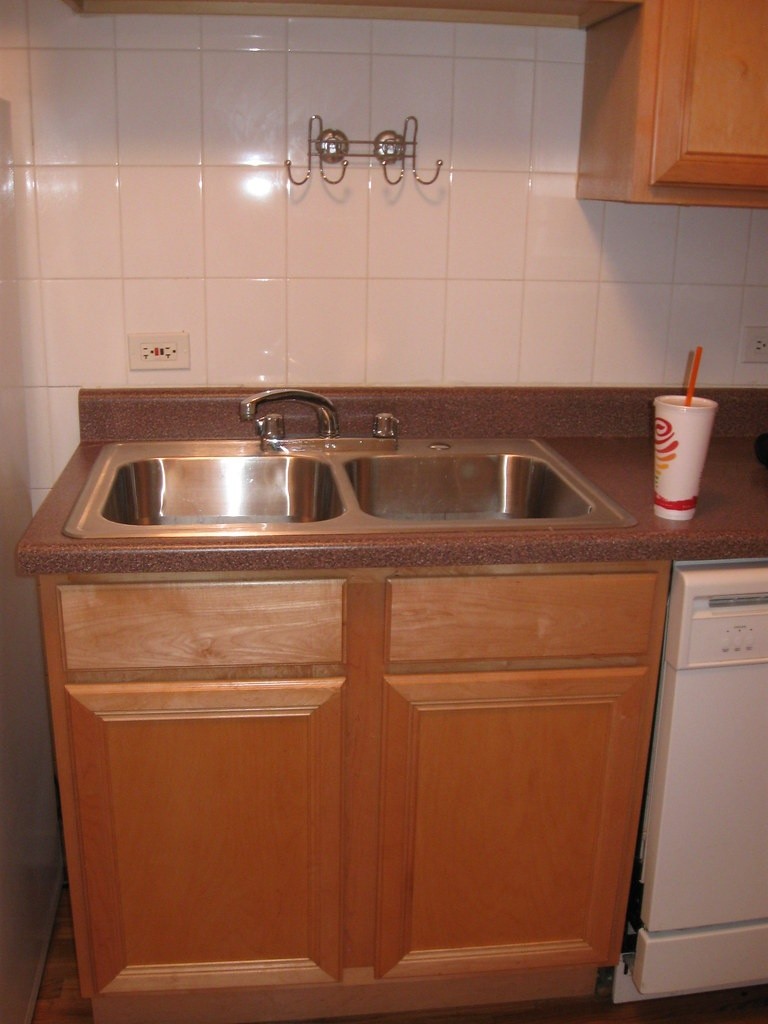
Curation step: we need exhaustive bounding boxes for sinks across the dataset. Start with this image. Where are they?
[341,452,598,534]
[62,454,348,537]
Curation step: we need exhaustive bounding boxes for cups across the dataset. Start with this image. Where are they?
[653,394,718,520]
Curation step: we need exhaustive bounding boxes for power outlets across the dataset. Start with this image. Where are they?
[126,330,191,371]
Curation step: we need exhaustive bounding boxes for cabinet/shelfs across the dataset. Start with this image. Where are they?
[37,559,671,1024]
[576,0,768,214]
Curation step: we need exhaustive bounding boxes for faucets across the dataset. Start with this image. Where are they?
[238,386,341,438]
